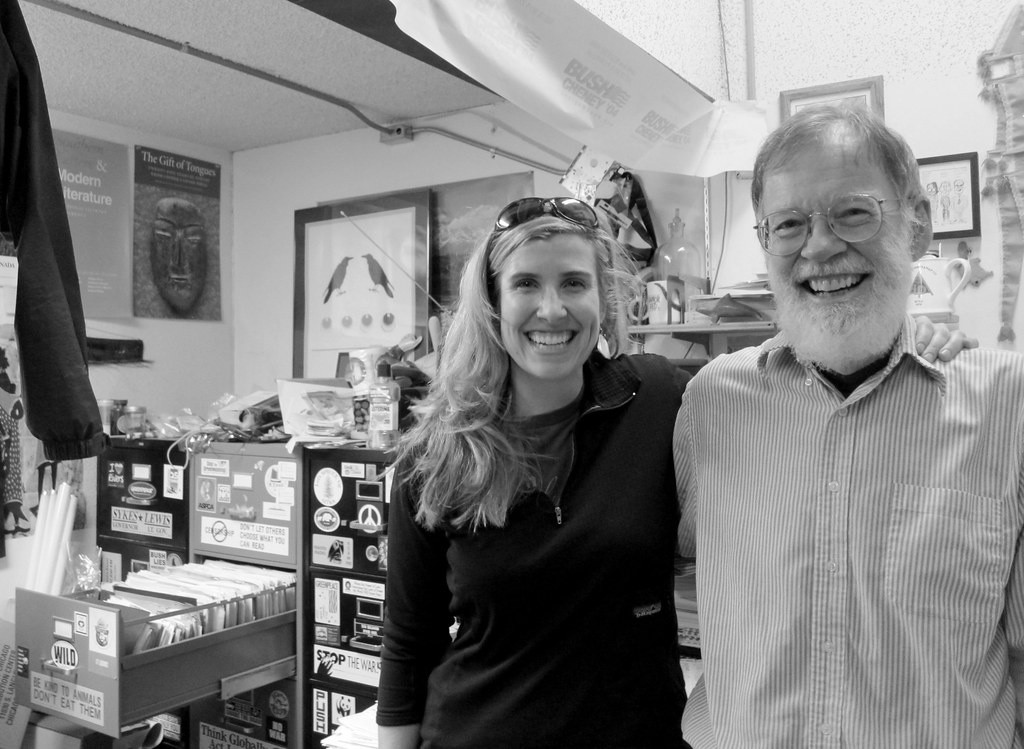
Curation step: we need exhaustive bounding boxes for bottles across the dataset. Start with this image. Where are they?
[366,365,401,449]
[653,210,703,322]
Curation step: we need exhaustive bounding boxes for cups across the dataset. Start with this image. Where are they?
[647,281,681,324]
[906,258,970,314]
[96,400,113,436]
[111,400,127,435]
[125,406,147,440]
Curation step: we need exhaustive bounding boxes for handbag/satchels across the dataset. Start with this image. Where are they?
[167,390,279,470]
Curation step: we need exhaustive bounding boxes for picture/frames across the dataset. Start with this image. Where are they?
[779,75,885,126]
[292,186,433,379]
[915,151,982,240]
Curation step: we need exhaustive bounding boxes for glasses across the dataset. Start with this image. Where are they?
[487,196,601,258]
[753,193,910,258]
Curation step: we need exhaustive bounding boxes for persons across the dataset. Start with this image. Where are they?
[673,101,1024,748]
[374,195,982,748]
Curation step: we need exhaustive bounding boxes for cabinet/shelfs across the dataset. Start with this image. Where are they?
[14,431,395,749]
